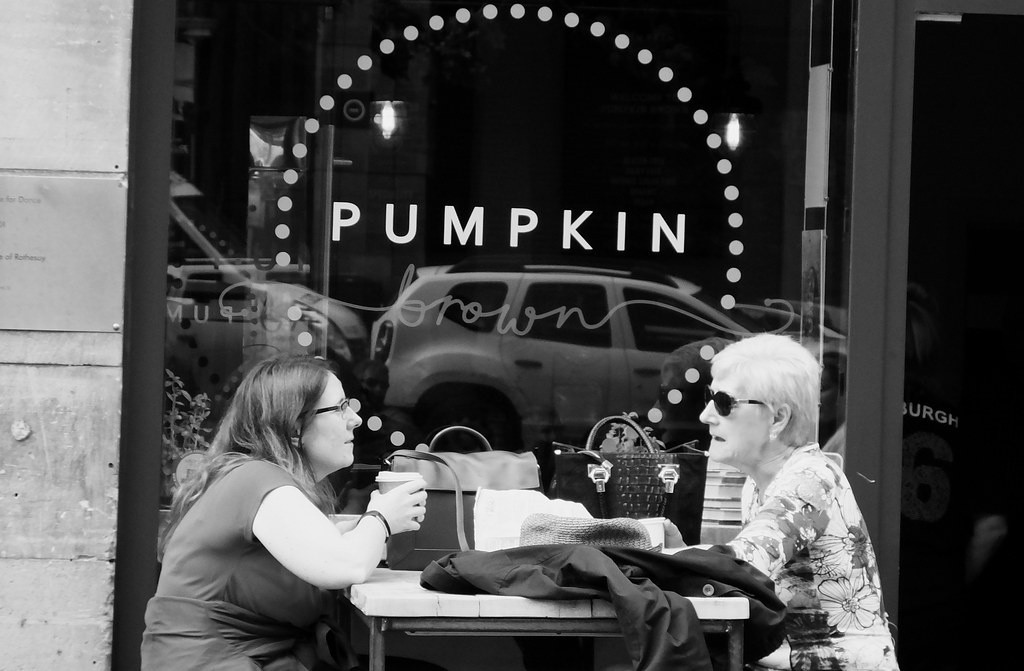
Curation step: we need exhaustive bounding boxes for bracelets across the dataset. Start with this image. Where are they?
[357,510,391,543]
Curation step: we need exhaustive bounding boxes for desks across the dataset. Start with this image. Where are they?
[339,565,751,671]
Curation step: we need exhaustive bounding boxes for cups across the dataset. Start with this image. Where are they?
[639,517,666,549]
[375,470,422,495]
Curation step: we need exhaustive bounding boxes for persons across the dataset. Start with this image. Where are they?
[353,357,390,408]
[661,331,901,671]
[813,352,846,467]
[140,356,428,671]
[654,336,737,446]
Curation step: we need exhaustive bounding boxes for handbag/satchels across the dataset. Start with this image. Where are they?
[553,416,709,546]
[384,426,544,571]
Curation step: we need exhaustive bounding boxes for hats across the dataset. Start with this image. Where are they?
[519,513,664,556]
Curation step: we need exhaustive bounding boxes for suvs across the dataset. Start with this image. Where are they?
[168,170,371,449]
[363,250,848,471]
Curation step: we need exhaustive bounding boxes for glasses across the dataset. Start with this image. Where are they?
[297,398,350,420]
[704,385,766,416]
[657,384,685,405]
